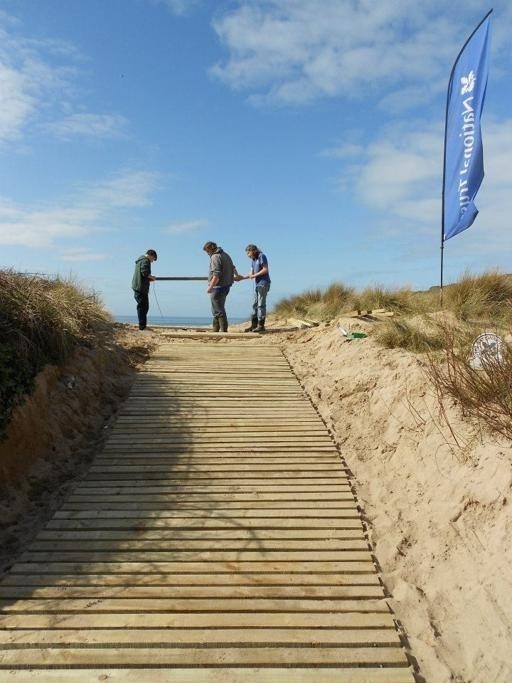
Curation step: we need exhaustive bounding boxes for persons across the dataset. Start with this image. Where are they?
[130,248,158,332]
[244,243,272,333]
[202,240,243,332]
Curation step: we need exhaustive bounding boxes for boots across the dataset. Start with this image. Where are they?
[212,317,228,332]
[253,319,266,332]
[245,318,258,332]
